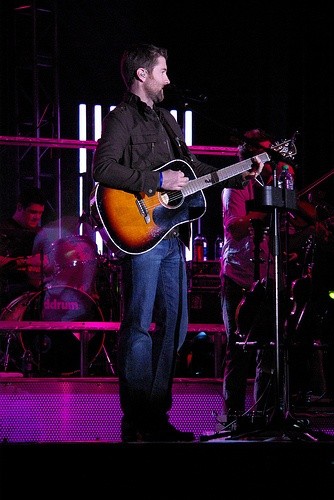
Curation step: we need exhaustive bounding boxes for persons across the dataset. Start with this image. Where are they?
[0,43,328,443]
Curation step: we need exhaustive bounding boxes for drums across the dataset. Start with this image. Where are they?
[0,292,37,360]
[46,234,99,296]
[21,287,107,375]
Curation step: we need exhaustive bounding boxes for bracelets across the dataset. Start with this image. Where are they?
[159,170,162,190]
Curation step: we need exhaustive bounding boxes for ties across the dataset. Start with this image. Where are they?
[157,110,188,161]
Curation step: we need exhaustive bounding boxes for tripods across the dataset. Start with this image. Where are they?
[226,165,334,441]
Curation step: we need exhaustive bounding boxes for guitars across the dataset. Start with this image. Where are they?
[87,134,297,250]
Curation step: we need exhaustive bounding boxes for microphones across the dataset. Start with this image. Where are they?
[168,84,207,102]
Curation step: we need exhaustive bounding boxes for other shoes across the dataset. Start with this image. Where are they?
[122,414,196,442]
